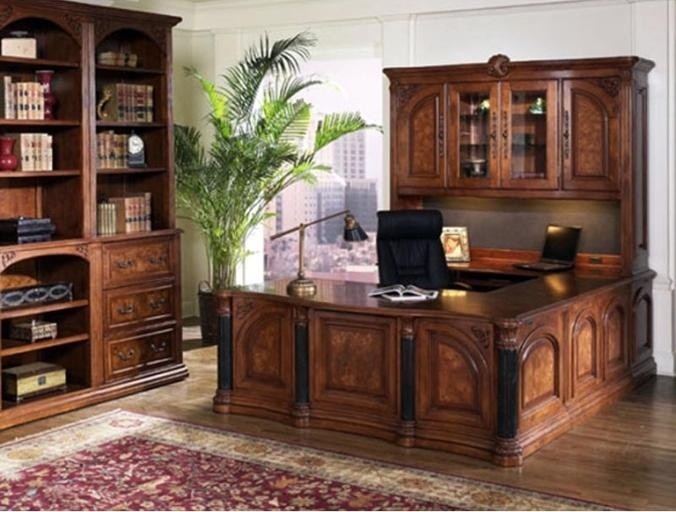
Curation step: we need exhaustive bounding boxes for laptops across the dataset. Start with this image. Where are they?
[511,223,582,273]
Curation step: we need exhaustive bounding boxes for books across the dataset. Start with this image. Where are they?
[367,283,441,303]
[97,50,152,235]
[3,75,54,172]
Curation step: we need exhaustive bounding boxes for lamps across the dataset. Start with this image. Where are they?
[270,209,368,296]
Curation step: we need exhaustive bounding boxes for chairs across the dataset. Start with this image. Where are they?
[376,210,453,289]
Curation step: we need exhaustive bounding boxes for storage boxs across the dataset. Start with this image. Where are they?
[2,361,65,403]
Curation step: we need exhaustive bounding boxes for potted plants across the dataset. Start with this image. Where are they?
[171,32,383,344]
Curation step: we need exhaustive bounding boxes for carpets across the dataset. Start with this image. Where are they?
[0,407,622,511]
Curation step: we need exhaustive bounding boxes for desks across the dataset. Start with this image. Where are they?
[213,248,657,466]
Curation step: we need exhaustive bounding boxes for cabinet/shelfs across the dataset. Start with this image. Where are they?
[447,54,558,199]
[383,63,446,197]
[0,0,190,430]
[558,56,655,200]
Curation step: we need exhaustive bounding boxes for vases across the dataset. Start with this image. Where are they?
[0,136,17,171]
[36,71,56,119]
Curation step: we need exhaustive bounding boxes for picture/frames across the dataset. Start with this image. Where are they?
[441,226,470,262]
